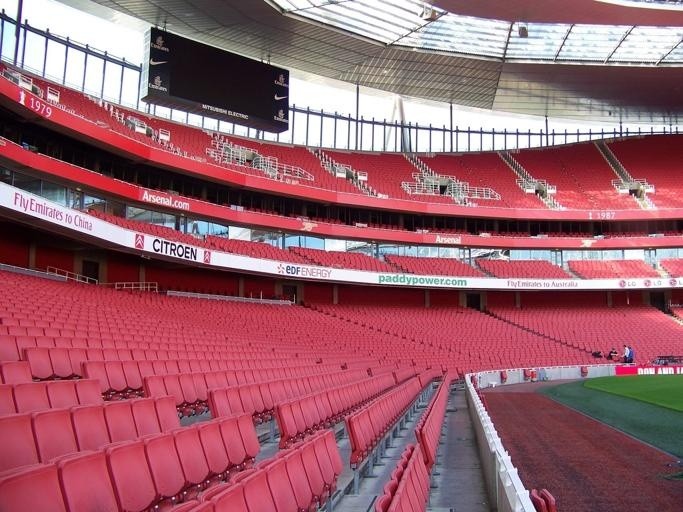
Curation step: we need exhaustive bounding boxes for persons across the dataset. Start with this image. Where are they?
[608,347,619,360]
[627,345,634,363]
[619,344,630,363]
